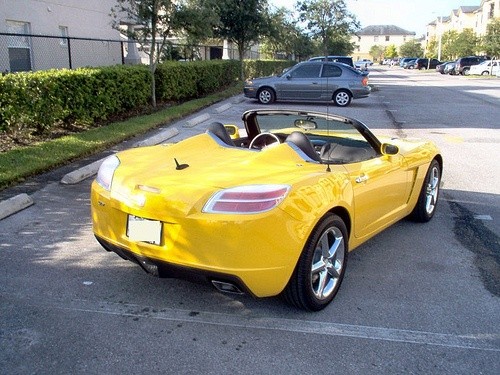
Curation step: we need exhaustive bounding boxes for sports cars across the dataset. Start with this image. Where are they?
[89,108,445,312]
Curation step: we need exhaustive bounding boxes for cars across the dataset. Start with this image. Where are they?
[469,59,500,76]
[308,55,373,72]
[382,57,458,75]
[242,59,373,107]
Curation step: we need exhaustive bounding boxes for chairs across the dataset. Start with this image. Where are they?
[207,122,236,146]
[284,131,323,164]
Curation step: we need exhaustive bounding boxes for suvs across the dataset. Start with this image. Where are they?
[455,55,491,76]
